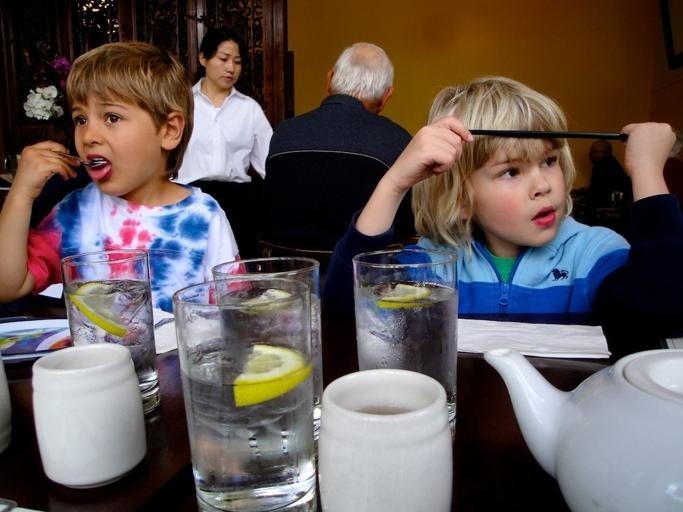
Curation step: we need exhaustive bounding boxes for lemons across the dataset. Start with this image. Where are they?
[241,288,293,311]
[70,282,127,338]
[233,344,313,407]
[382,284,431,302]
[378,300,436,309]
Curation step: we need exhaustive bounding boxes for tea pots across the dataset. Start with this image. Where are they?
[482,342,683,508]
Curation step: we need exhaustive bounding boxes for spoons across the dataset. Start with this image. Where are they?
[45,152,108,170]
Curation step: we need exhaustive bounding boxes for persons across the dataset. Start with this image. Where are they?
[168,27,274,260]
[320,75,680,343]
[266,43,413,165]
[570,137,632,227]
[0,41,254,350]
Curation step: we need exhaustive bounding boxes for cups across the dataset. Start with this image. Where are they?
[350,252,460,421]
[60,250,165,416]
[169,257,325,506]
[319,366,453,508]
[33,342,148,489]
